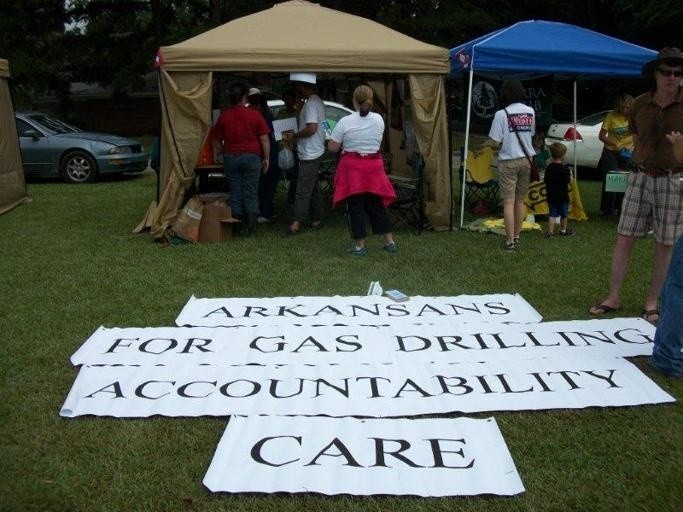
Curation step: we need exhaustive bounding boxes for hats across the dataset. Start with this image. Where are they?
[248,87,264,96]
[643,47,683,77]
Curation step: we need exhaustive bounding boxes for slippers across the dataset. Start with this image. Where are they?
[588,302,622,316]
[640,308,660,324]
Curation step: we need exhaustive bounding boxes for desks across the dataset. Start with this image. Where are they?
[196,163,234,213]
[601,171,633,218]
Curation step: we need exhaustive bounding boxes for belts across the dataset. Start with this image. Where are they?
[637,166,683,177]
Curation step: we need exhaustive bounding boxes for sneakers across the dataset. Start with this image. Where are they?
[234,212,397,255]
[500,229,577,252]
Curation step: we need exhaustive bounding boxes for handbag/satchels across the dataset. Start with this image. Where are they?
[279,143,295,170]
[174,199,203,242]
[531,161,539,182]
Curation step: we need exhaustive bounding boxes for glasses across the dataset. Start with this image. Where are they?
[657,66,683,76]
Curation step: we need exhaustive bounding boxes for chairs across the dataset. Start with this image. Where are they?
[456,146,499,214]
[321,147,427,239]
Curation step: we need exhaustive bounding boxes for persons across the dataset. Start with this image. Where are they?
[597,92,635,217]
[585,47,683,320]
[652,230,683,379]
[489,75,536,247]
[546,143,573,236]
[212,77,398,257]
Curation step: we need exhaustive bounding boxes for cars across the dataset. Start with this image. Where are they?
[240,99,355,167]
[15,111,147,183]
[544,106,614,173]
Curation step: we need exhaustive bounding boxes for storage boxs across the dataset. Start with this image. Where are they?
[201,203,242,241]
